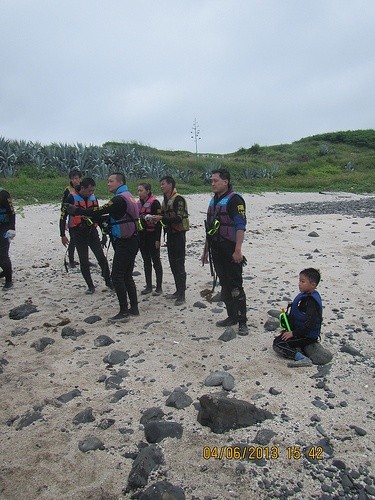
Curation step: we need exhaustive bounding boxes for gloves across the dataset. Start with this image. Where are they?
[5,229,16,239]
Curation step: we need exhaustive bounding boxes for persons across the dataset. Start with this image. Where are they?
[146,176,189,306]
[59,177,115,295]
[273,267,323,367]
[201,169,249,336]
[64,173,140,323]
[135,183,162,296]
[61,169,95,269]
[0,188,16,291]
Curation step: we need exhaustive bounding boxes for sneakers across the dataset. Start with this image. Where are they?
[166,293,179,298]
[152,288,163,296]
[106,281,115,291]
[238,321,249,335]
[216,317,238,327]
[141,288,152,295]
[109,312,130,322]
[175,296,185,305]
[2,281,13,291]
[84,288,95,294]
[287,356,312,367]
[127,309,140,316]
